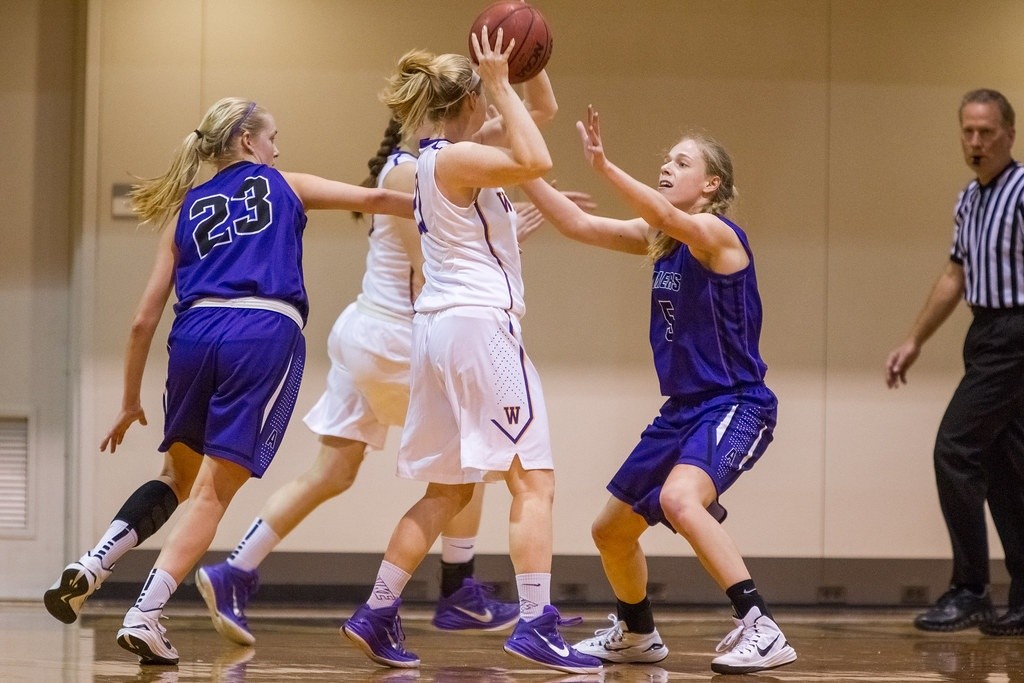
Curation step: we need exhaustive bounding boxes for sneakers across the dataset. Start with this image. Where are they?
[194,559,259,645]
[913,586,997,631]
[339,598,421,667]
[117,606,180,665]
[502,605,603,674]
[43,550,114,624]
[711,605,798,674]
[571,614,669,663]
[978,607,1024,636]
[430,578,521,632]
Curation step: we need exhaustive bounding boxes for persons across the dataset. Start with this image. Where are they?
[339,24,603,674]
[886,89,1023,636]
[195,50,598,645]
[42,95,414,662]
[486,103,797,674]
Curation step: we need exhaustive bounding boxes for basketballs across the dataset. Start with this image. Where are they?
[466,0,554,87]
